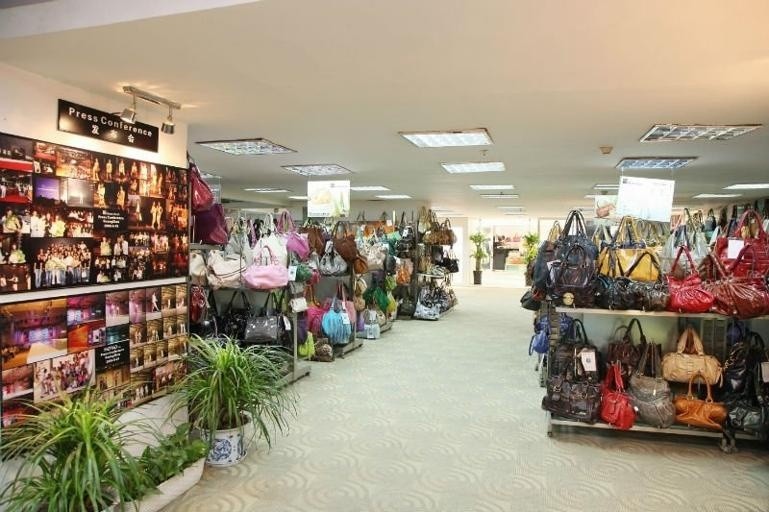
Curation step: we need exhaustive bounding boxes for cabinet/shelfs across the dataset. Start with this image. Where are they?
[546,305,769,439]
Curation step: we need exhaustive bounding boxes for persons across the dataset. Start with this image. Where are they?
[1,130,197,456]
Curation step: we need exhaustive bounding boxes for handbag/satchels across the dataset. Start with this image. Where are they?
[189,205,357,362]
[521,216,769,320]
[529,315,768,434]
[597,204,615,218]
[356,216,459,331]
[192,172,212,213]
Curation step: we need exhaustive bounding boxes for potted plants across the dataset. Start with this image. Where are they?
[124,424,207,512]
[162,331,303,468]
[0,373,166,512]
[469,232,488,284]
[523,234,539,286]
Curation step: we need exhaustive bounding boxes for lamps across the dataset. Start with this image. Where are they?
[120,85,180,134]
